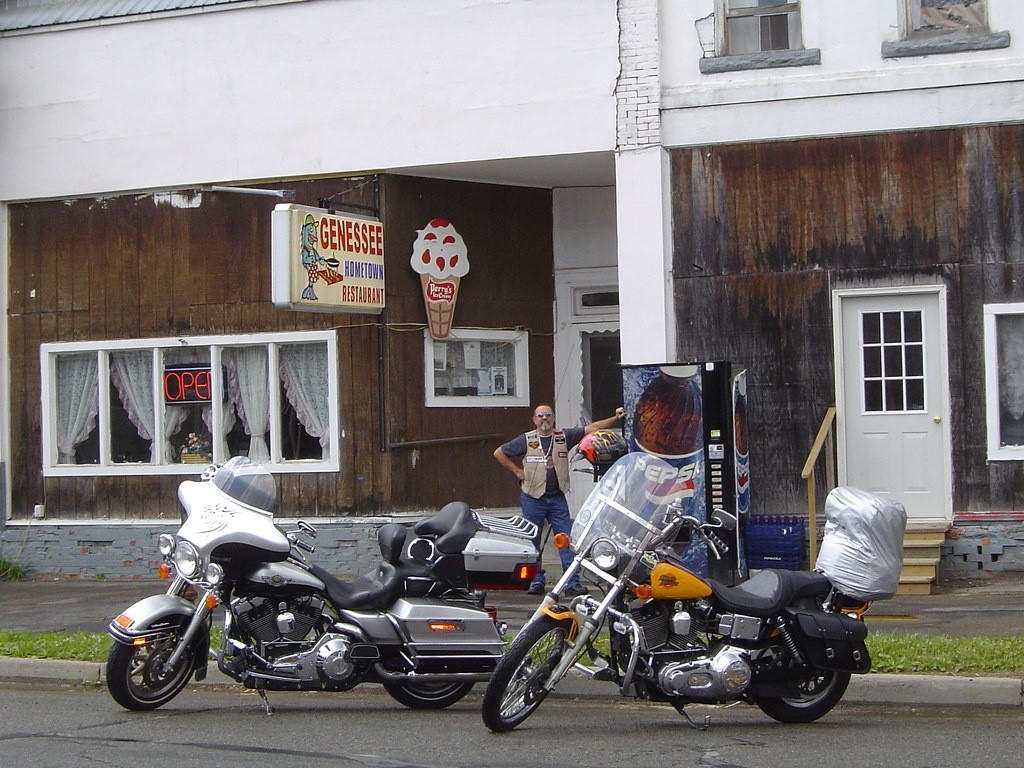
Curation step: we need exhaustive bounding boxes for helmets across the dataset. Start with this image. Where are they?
[570,429,629,465]
[200,464,235,493]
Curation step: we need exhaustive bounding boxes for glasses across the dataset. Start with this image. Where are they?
[536,413,552,417]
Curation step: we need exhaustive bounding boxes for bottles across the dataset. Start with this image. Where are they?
[736,373,750,534]
[630,365,700,556]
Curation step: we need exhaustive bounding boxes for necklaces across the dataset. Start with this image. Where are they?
[537,431,553,465]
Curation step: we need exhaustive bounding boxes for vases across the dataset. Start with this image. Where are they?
[180,454,210,464]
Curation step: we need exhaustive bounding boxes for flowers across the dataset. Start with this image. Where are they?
[179,433,213,460]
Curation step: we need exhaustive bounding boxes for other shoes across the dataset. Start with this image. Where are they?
[527,584,544,594]
[565,584,588,596]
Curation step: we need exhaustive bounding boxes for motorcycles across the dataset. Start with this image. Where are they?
[105,454,543,712]
[483,452,905,733]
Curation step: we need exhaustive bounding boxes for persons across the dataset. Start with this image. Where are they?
[492,405,626,597]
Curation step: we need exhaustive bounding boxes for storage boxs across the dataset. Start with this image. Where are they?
[748,516,805,570]
